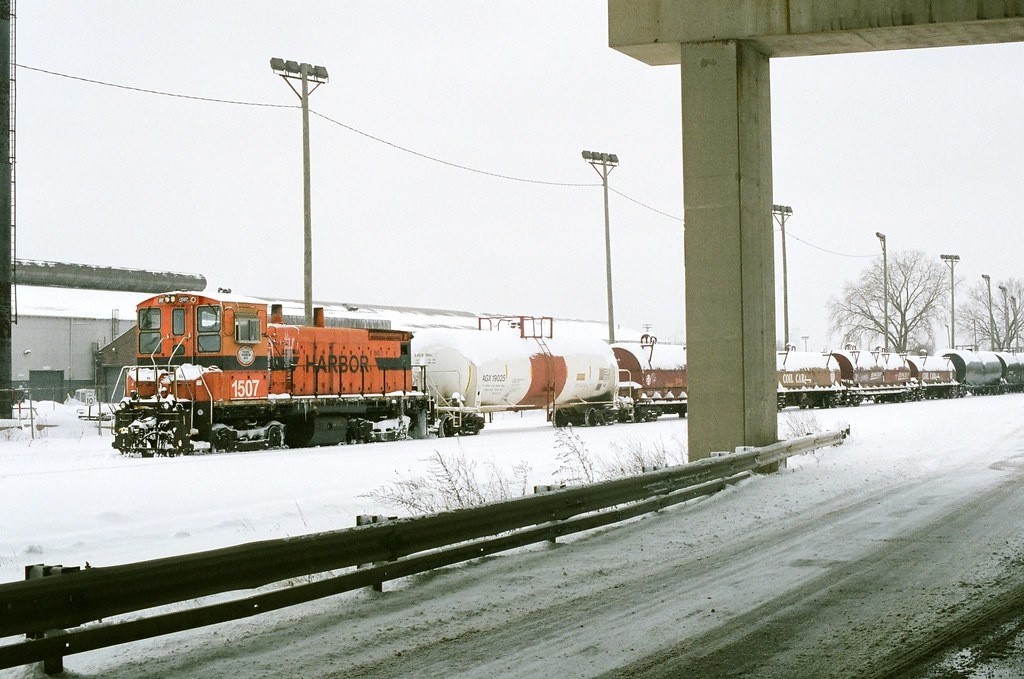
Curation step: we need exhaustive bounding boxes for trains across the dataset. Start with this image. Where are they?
[108,290,1023,459]
